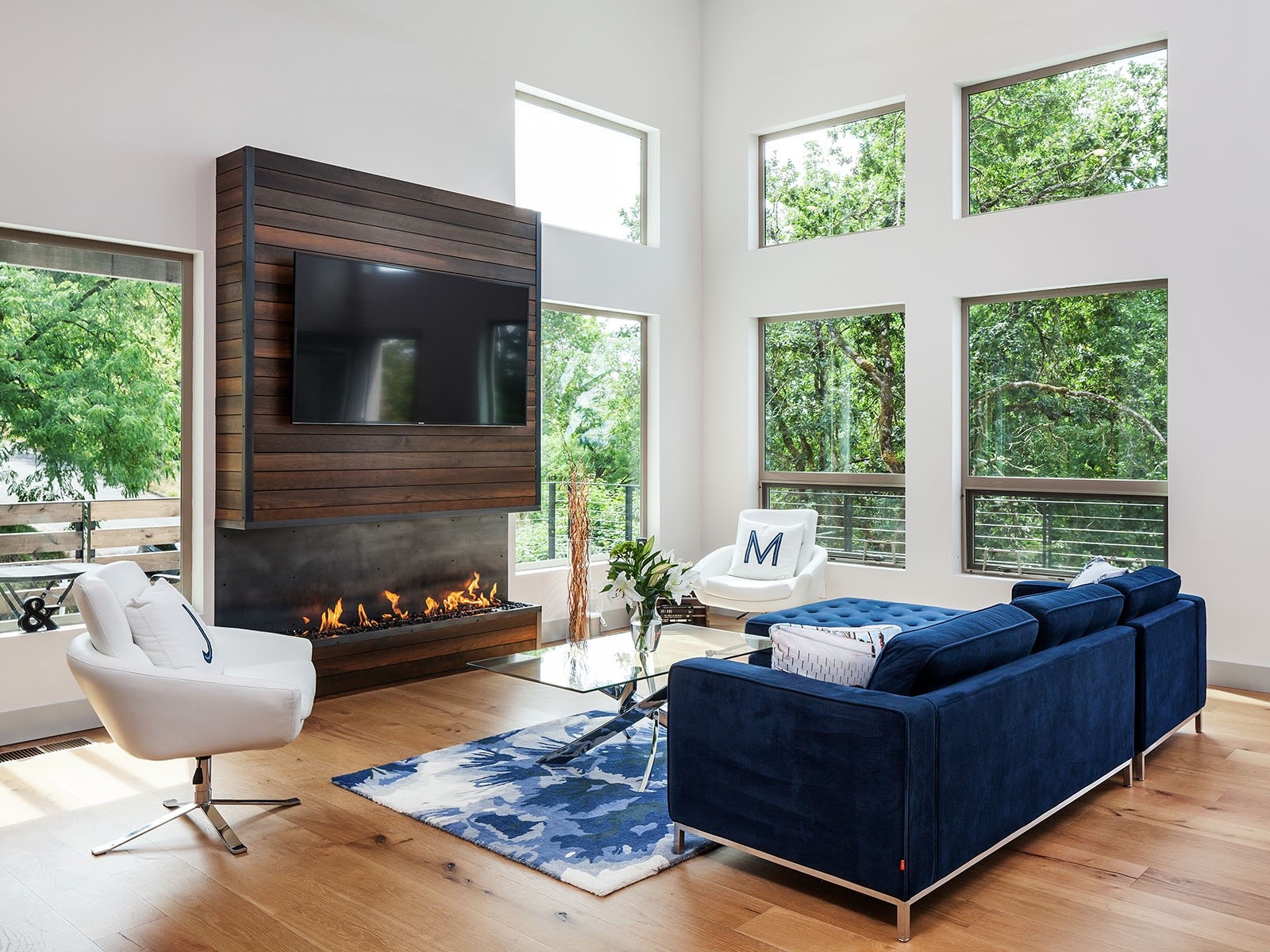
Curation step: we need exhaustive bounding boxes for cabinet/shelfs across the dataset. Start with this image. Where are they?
[656,591,710,629]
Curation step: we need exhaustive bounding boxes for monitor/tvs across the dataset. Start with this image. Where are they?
[290,251,530,428]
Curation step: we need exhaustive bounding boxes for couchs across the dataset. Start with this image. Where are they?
[667,565,1206,941]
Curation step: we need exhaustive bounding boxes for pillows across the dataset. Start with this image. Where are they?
[124,577,221,674]
[728,516,805,580]
[767,619,901,689]
[1067,556,1128,589]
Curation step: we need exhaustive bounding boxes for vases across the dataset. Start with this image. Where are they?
[629,596,662,652]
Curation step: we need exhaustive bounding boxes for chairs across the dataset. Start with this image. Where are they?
[691,508,829,615]
[66,560,316,855]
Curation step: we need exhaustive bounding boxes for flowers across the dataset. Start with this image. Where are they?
[600,536,701,648]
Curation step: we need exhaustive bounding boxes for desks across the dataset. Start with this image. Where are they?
[468,624,771,793]
[0,563,102,633]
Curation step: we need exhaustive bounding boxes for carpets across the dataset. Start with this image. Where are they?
[330,696,720,897]
